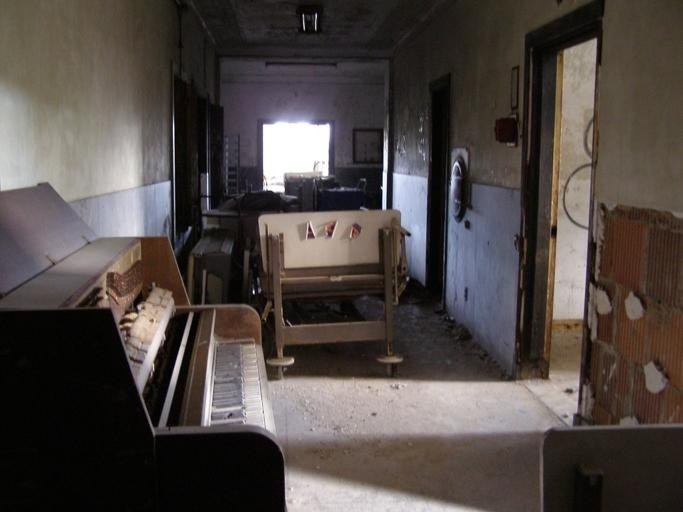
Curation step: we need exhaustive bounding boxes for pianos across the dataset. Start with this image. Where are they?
[1,181,287,512]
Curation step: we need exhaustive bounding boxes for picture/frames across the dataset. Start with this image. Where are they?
[352,128,383,164]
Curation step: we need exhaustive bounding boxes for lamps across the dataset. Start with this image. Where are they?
[296,7,322,33]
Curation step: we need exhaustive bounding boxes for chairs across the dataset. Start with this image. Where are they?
[539,424,682,512]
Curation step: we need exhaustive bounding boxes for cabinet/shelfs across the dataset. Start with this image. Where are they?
[206,171,367,256]
